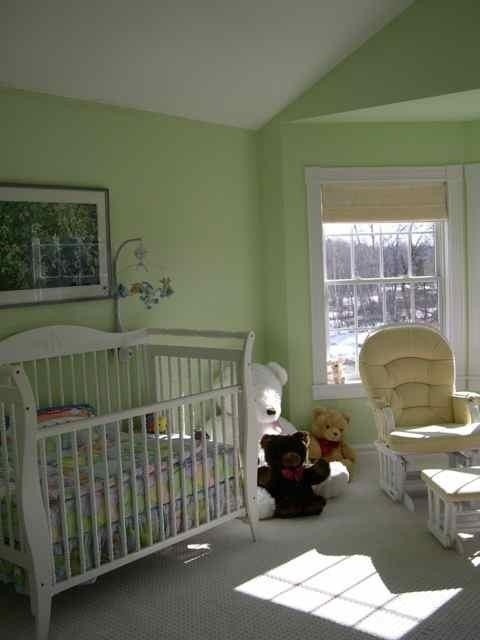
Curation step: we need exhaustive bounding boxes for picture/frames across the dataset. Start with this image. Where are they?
[0,182,112,309]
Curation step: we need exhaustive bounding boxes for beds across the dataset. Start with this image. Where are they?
[0,323,258,640]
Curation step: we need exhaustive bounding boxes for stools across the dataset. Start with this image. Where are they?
[419,466,480,550]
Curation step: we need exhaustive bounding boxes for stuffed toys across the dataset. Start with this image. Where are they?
[307,405,357,476]
[145,415,169,433]
[255,430,332,517]
[202,362,350,522]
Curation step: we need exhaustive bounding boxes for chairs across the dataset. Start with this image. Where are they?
[358,321,480,504]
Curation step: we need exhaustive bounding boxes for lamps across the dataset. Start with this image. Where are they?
[112,234,175,333]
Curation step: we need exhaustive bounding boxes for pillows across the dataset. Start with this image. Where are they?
[1,403,106,453]
